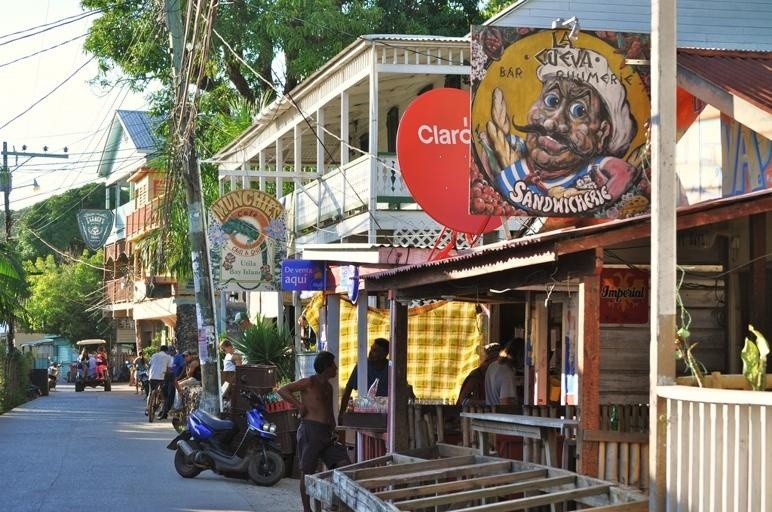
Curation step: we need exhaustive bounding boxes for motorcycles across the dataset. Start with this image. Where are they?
[46,356,63,392]
[135,365,150,397]
[165,389,287,489]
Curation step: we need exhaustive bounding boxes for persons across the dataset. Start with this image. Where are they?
[480,335,527,414]
[75,339,242,423]
[275,350,353,511]
[334,335,412,423]
[454,340,500,413]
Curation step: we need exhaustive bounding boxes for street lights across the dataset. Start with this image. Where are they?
[4,177,41,243]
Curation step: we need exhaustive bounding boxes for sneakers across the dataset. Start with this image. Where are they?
[172,407,184,413]
[158,411,167,420]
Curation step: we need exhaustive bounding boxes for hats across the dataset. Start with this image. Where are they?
[167,346,175,354]
[233,312,247,323]
[183,351,191,355]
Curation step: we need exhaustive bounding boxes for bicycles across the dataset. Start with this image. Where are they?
[144,376,167,424]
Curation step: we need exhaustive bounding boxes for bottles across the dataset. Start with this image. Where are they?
[610,405,618,431]
[346,377,455,414]
[262,388,295,413]
